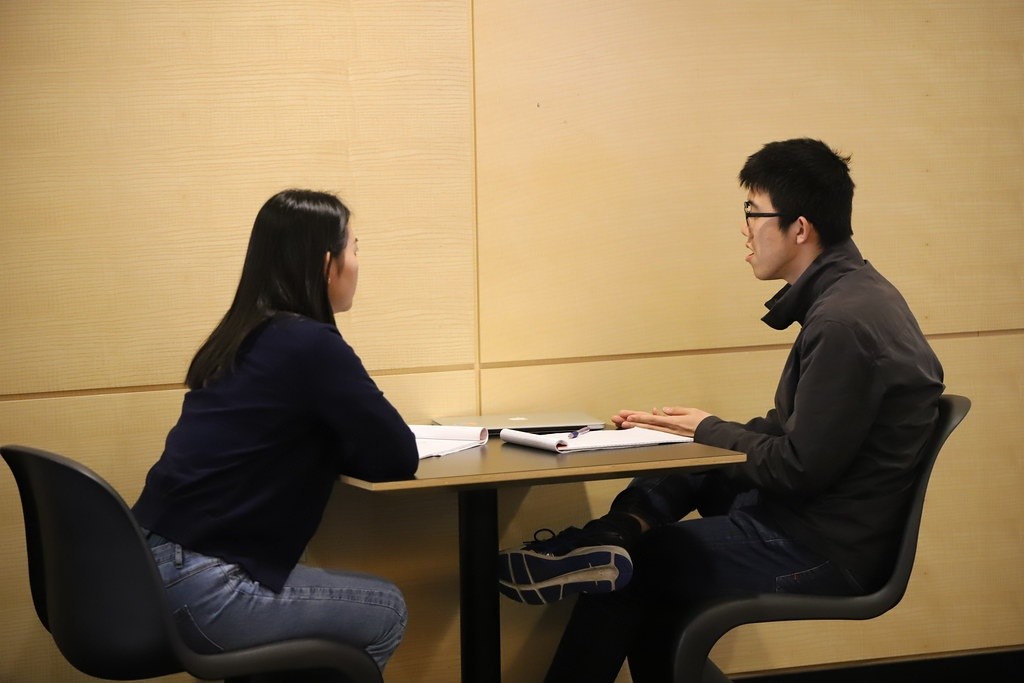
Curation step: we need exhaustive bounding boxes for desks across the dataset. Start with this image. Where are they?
[338,423,747,683]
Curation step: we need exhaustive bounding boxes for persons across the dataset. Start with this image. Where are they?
[494,137,946,683]
[128,187,412,683]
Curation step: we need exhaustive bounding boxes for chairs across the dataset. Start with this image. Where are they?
[1,444,387,683]
[668,395,971,683]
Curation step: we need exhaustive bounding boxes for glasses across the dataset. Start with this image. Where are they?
[742,202,791,230]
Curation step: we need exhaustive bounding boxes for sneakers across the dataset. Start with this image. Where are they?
[502,523,632,606]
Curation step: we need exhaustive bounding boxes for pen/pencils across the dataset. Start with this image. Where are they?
[567,426,591,439]
